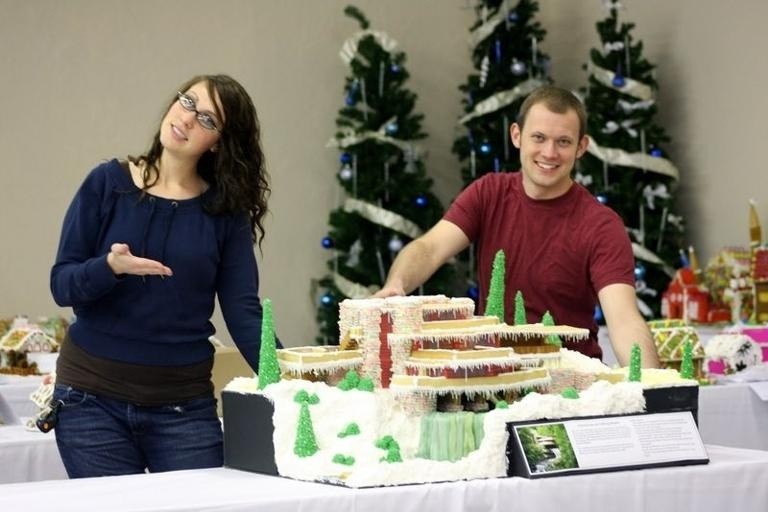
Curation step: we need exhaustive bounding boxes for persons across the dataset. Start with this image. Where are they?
[47,75,280,480]
[369,84,664,373]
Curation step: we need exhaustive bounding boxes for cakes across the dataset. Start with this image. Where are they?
[1,316,68,374]
[223,249,647,487]
[650,201,768,383]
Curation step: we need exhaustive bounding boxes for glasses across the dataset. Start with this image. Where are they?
[177,88,223,140]
